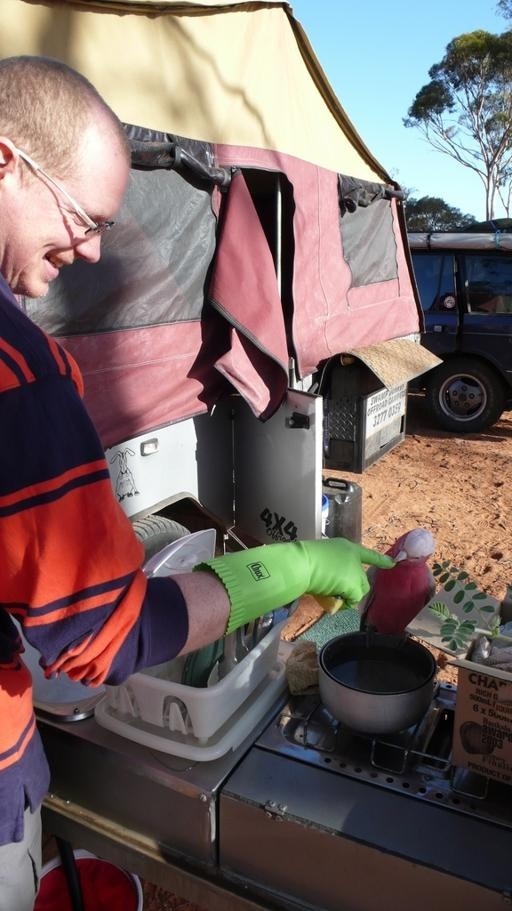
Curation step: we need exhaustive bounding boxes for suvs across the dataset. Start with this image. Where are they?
[406,220,512,431]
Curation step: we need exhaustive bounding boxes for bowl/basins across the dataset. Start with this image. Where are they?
[316,629,436,734]
[33,849,148,911]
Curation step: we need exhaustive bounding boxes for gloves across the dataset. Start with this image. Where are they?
[188,534,396,642]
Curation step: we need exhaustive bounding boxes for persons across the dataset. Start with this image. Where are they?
[0,52,396,910]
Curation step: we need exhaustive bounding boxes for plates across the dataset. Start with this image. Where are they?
[136,524,290,686]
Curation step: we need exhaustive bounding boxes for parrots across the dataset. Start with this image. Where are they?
[356,526,441,649]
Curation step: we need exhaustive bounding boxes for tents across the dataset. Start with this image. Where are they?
[0,2,443,454]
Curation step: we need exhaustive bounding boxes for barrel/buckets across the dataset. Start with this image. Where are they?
[322,493,330,538]
[322,476,362,543]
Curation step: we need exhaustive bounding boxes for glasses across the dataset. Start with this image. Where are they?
[16,147,115,240]
